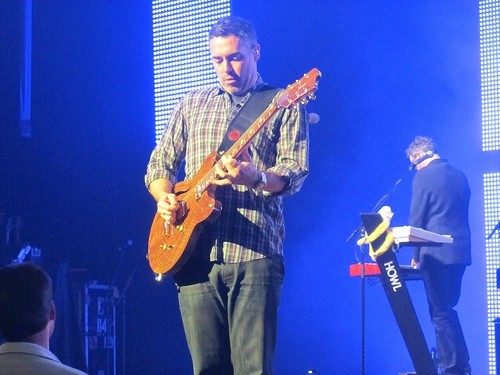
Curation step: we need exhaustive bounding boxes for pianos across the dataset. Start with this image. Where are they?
[365,225,454,244]
[349,262,439,375]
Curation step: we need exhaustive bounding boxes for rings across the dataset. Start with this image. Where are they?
[164,212,165,214]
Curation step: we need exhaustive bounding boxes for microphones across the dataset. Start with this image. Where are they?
[118,240,132,251]
[410,150,433,169]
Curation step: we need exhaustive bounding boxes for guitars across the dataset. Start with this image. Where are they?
[147,68,321,276]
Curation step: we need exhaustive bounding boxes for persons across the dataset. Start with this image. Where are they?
[405,136,471,375]
[0,263,88,375]
[145,16,309,375]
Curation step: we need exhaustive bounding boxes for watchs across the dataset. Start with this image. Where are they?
[255,172,267,190]
